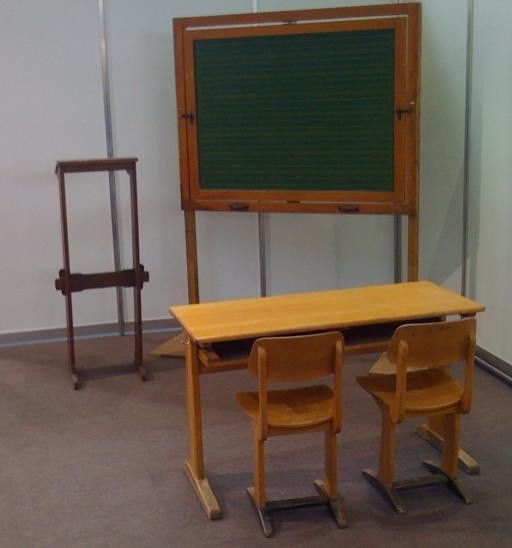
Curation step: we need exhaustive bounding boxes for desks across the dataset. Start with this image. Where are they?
[166,277,486,529]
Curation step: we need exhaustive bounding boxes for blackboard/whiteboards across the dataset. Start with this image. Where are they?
[183,16,406,202]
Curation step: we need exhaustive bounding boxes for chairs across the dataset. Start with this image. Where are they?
[237,329,348,535]
[355,321,479,516]
